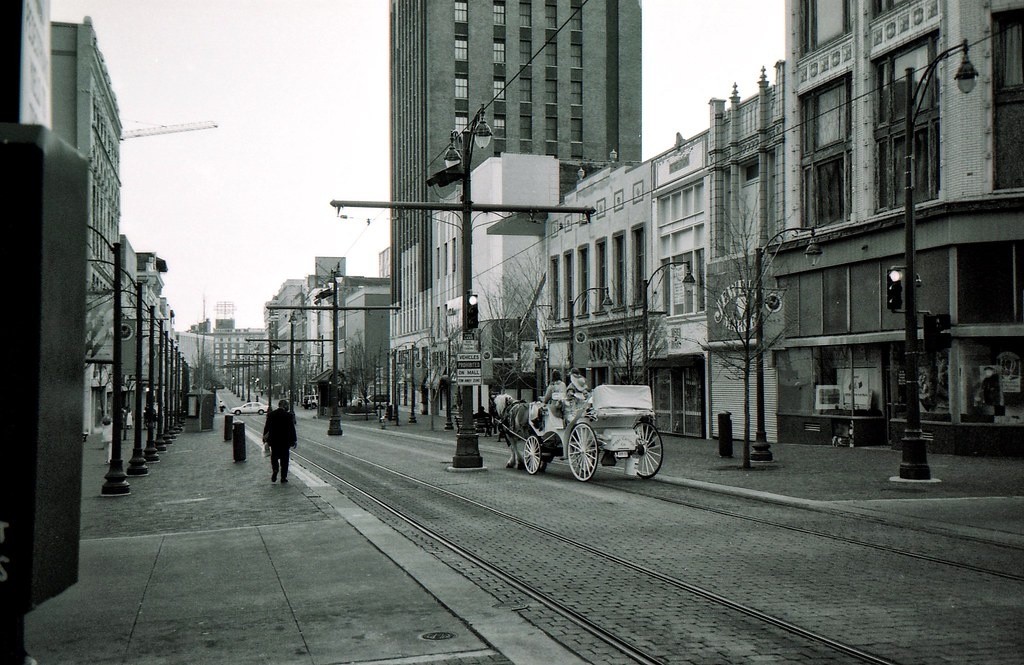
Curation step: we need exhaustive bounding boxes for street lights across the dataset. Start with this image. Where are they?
[642,261,696,447]
[441,101,492,468]
[566,286,615,385]
[898,37,981,481]
[288,310,299,415]
[408,336,438,423]
[391,341,417,419]
[325,260,343,436]
[749,223,823,461]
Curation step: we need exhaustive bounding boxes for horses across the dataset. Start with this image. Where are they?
[489,393,566,473]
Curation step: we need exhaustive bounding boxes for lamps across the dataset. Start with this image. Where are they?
[609,148,617,163]
[577,167,585,181]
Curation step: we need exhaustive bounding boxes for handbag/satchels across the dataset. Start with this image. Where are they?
[262,442,272,458]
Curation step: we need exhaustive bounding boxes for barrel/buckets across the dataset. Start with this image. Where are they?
[624,455,640,476]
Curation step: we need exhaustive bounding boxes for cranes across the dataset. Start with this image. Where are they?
[119,116,220,142]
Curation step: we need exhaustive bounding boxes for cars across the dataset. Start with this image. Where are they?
[230,401,268,415]
[347,392,391,410]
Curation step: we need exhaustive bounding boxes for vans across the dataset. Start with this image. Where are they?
[302,395,319,409]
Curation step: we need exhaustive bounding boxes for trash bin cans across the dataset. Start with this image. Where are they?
[224,415,233,440]
[718,410,734,458]
[232,422,246,463]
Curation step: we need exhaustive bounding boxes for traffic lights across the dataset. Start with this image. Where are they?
[465,293,479,329]
[886,269,903,310]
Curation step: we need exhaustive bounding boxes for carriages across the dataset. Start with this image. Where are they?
[487,383,664,483]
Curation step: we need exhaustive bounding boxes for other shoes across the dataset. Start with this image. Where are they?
[281,478,288,483]
[272,470,277,482]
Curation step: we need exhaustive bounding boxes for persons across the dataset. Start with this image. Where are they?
[489,397,498,435]
[304,397,308,404]
[474,406,492,436]
[538,371,566,404]
[121,406,132,428]
[143,404,157,430]
[101,414,112,463]
[219,400,224,412]
[982,367,1001,404]
[263,399,297,483]
[566,368,588,393]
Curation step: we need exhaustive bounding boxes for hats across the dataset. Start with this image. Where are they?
[571,373,587,391]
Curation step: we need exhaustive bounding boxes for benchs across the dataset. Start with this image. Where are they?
[548,391,586,430]
[567,384,652,429]
